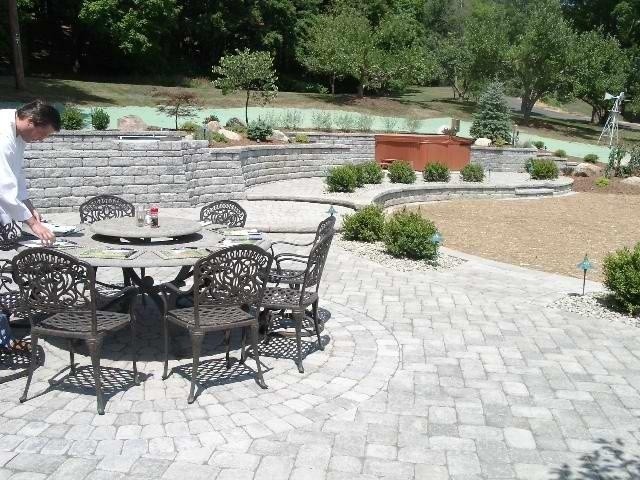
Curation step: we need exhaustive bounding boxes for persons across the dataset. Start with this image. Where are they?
[0,99,62,352]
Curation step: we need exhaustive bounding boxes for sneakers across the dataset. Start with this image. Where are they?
[3,339,31,355]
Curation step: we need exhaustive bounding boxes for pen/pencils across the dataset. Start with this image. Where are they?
[182,247,197,249]
[125,249,137,258]
[206,248,214,254]
[68,241,78,245]
[218,238,225,243]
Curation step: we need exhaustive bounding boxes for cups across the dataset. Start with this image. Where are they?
[136,209,147,227]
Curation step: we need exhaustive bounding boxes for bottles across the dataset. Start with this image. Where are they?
[150,208,158,227]
[143,202,153,225]
